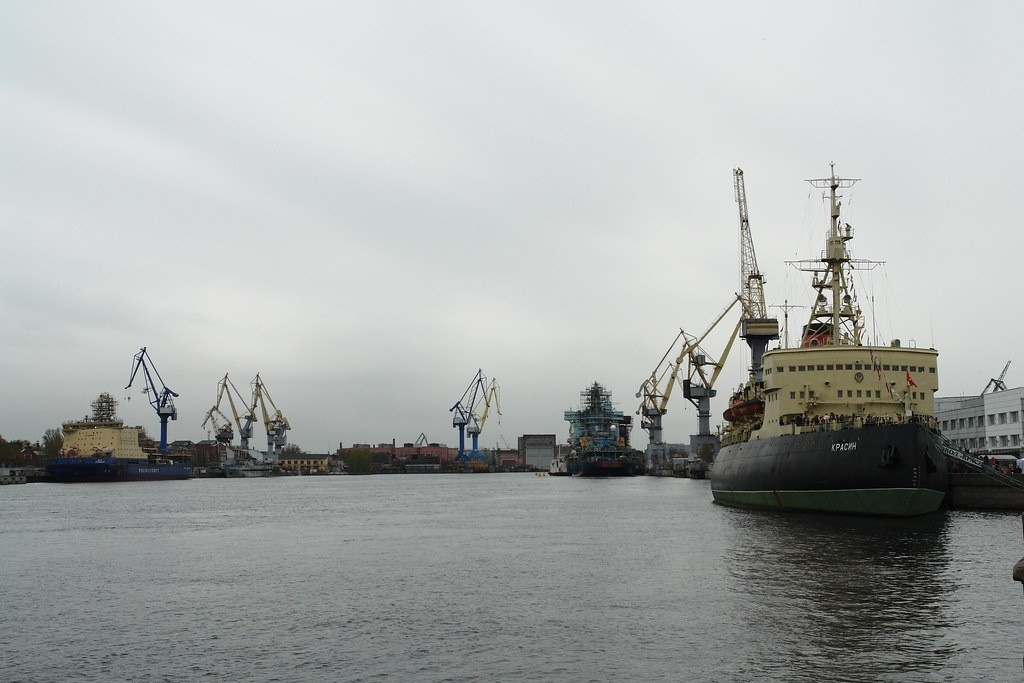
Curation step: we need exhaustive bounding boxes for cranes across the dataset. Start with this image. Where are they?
[124,347,290,462]
[449,368,502,458]
[633,293,748,464]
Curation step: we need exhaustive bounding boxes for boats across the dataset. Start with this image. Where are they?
[563,380,645,477]
[42,392,192,481]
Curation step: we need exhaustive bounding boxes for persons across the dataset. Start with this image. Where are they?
[779,413,941,436]
[958,446,1022,474]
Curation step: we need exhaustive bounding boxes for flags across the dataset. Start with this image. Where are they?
[908,372,917,388]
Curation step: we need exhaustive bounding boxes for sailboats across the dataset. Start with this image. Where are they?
[711,160,948,518]
[548,441,572,477]
